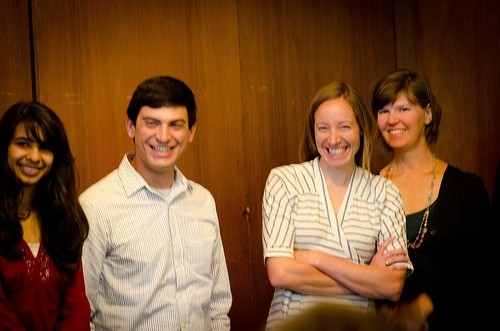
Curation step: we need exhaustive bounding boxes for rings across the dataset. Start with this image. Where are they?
[385,260,389,265]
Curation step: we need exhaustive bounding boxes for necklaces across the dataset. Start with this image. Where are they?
[385,154,438,249]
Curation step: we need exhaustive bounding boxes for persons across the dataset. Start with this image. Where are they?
[263,300,408,331]
[262,80,413,331]
[77,76,233,331]
[371,68,500,331]
[0,101,91,331]
